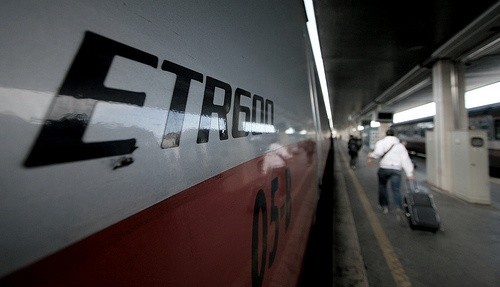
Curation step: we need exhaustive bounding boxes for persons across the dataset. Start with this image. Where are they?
[368,130,414,214]
[347,134,362,170]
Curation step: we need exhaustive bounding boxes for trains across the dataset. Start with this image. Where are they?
[1,0,338,287]
[385,103,500,167]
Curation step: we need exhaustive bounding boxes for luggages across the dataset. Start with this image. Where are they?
[403,193,439,234]
